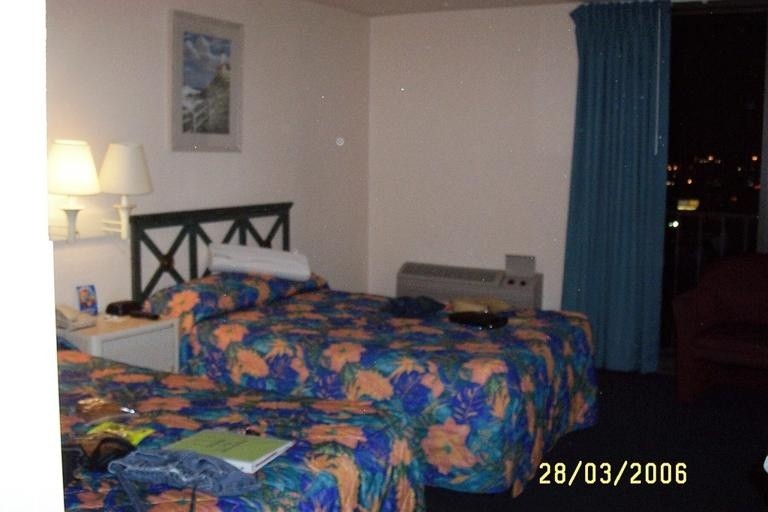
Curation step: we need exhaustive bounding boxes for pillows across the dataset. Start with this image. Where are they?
[142,242,328,333]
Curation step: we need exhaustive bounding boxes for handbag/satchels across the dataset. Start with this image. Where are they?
[450,297,514,314]
[108,448,259,497]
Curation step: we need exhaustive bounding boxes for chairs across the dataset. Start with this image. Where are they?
[673,253,768,403]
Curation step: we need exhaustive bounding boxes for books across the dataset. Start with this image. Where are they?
[88,421,155,447]
[163,428,297,475]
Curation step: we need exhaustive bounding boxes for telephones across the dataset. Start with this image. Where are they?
[55,303,97,331]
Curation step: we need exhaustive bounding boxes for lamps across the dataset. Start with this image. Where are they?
[46,138,151,245]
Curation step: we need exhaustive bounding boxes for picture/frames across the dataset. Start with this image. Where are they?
[167,8,245,155]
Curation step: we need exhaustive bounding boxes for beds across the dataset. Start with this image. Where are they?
[128,200,589,493]
[56,334,426,511]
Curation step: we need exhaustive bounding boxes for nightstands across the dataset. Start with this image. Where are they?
[55,311,180,376]
